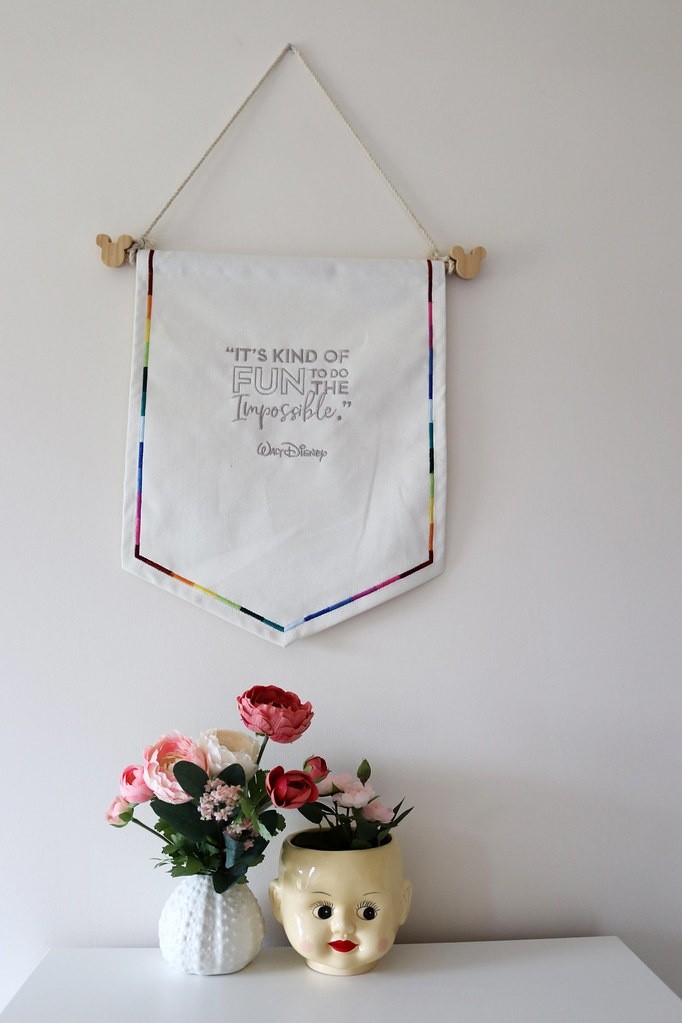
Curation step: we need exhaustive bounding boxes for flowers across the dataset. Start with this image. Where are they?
[105,685,331,893]
[297,759,415,849]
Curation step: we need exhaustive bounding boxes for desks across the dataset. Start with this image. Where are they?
[0,935,682,1023]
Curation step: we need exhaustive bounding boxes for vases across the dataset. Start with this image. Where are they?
[269,826,413,975]
[159,872,265,977]
